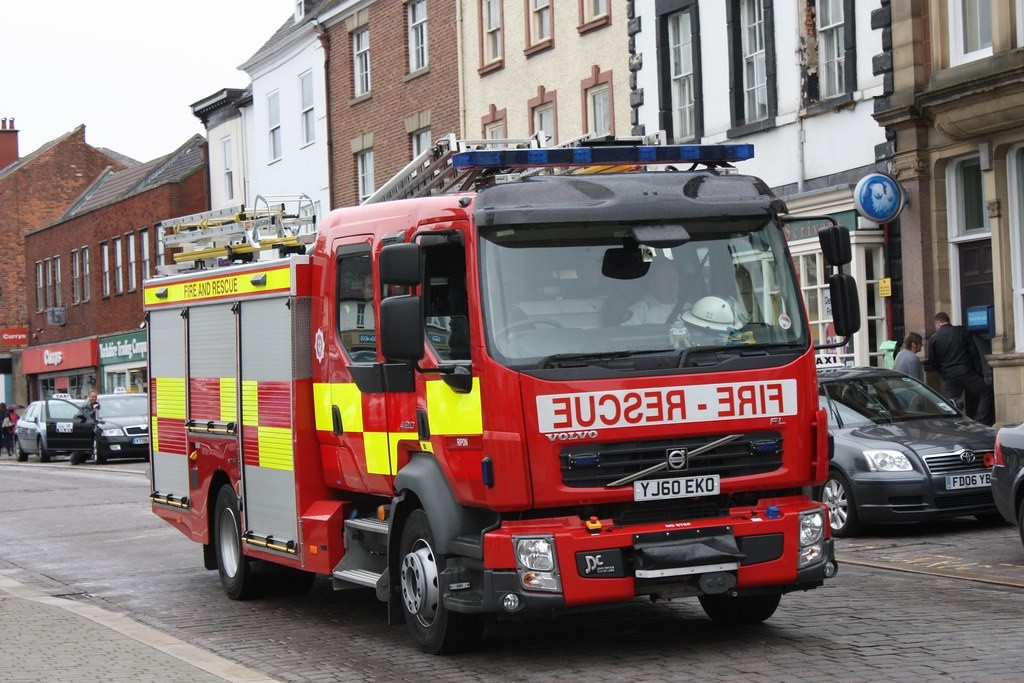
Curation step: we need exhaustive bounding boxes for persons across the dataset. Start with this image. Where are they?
[929,312,993,423]
[0,402,19,456]
[71,391,97,464]
[892,333,924,383]
[619,269,693,326]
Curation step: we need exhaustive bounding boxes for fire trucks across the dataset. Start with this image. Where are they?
[138,131,863,658]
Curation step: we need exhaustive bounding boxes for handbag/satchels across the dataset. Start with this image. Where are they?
[2,417,14,428]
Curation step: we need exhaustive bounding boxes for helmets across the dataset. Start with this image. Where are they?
[680,296,742,331]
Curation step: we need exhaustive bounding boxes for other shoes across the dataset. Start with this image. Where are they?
[8,452,12,456]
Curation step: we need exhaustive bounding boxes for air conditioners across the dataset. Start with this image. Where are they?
[47,307,65,326]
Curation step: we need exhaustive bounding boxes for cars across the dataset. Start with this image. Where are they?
[988,422,1024,549]
[807,364,999,538]
[11,393,87,464]
[83,385,149,465]
[51,391,88,412]
